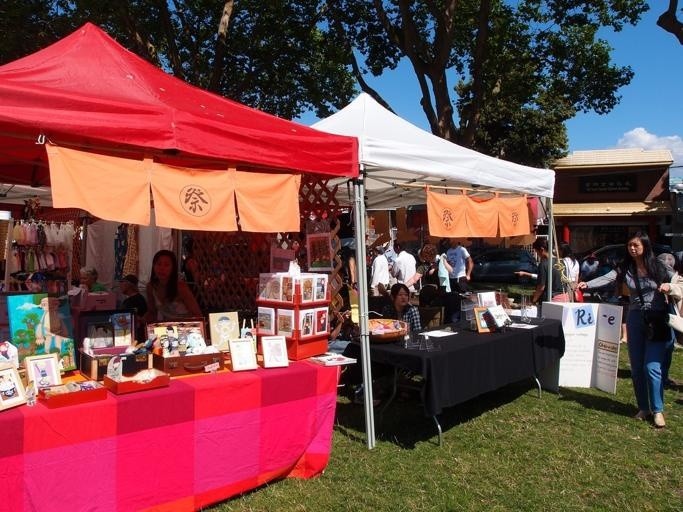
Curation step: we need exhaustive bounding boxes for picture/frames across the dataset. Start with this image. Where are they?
[228,339,259,371]
[96,304,114,310]
[262,335,289,368]
[306,232,334,272]
[23,353,62,394]
[270,247,295,274]
[0,361,28,412]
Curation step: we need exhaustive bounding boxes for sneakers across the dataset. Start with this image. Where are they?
[654,413,666,426]
[635,409,650,420]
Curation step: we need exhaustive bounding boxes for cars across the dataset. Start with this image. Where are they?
[562,241,676,277]
[422,246,539,284]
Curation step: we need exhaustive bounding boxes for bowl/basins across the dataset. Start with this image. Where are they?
[366,318,406,343]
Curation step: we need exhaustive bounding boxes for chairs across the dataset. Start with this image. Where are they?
[366,296,394,320]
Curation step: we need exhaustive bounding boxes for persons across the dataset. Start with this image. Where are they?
[328,239,474,391]
[512,241,599,304]
[186,239,307,300]
[578,232,682,427]
[119,272,148,316]
[77,266,108,292]
[146,252,201,328]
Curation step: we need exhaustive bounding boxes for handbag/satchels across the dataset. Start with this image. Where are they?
[644,310,671,342]
[574,289,582,302]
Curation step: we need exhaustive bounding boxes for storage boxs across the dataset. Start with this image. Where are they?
[145,316,224,376]
[76,307,154,380]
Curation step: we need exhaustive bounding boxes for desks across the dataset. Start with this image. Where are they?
[343,314,566,446]
[0,351,345,512]
